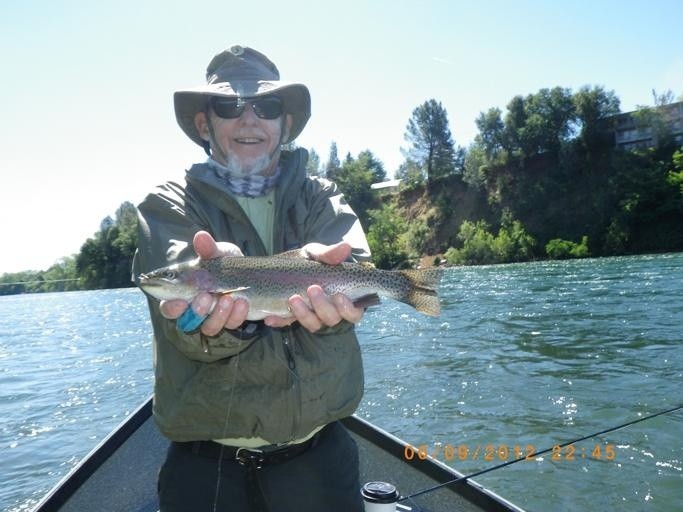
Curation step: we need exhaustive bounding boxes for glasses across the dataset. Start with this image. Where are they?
[206,95,284,120]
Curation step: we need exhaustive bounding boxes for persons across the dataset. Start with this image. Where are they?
[128,42,379,510]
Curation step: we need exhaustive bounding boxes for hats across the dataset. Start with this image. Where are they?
[171,45,312,147]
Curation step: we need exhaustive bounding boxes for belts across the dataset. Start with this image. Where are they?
[205,433,325,464]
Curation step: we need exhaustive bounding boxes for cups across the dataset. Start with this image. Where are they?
[361,479,401,512]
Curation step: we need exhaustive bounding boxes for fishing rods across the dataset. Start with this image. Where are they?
[396,405,682,502]
